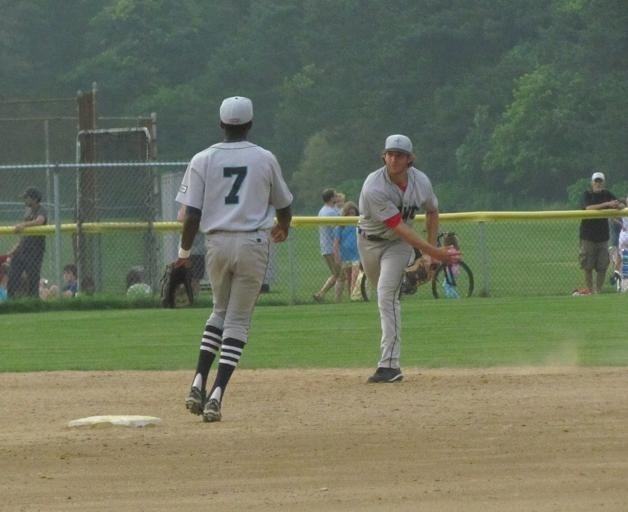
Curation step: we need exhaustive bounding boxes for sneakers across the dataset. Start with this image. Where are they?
[313,292,323,304]
[186,387,206,416]
[368,368,403,383]
[202,399,221,422]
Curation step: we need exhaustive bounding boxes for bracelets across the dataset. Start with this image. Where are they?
[176,247,191,259]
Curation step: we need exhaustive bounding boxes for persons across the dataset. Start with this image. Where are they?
[172,96,294,422]
[312,188,343,303]
[357,134,461,384]
[8,187,48,298]
[575,172,625,296]
[334,200,363,302]
[334,193,345,208]
[616,197,628,291]
[0,258,98,299]
[609,206,620,284]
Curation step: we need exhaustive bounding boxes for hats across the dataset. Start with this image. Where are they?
[18,187,41,201]
[383,135,412,155]
[592,172,605,184]
[220,96,253,125]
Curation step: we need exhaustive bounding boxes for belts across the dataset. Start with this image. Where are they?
[356,226,388,241]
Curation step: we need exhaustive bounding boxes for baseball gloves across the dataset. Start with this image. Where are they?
[161,257,195,308]
[400,254,442,295]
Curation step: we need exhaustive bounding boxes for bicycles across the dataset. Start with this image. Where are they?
[361,231,473,300]
